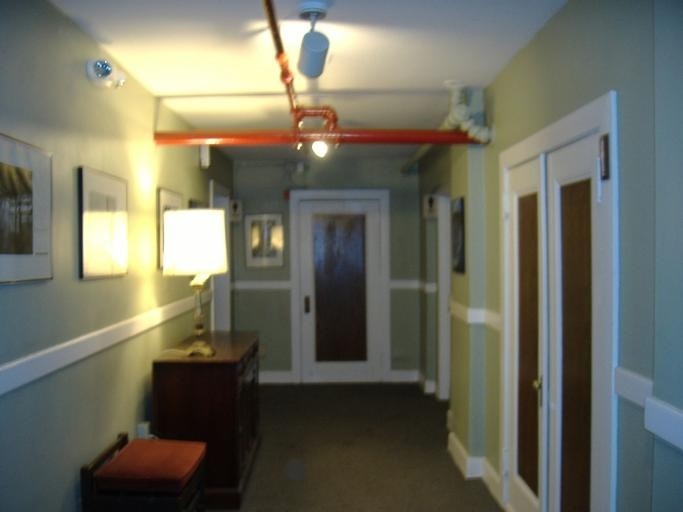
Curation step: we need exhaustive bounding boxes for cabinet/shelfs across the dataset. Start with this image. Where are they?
[152,329,261,511]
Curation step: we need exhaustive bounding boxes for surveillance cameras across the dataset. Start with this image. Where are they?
[87,58,112,81]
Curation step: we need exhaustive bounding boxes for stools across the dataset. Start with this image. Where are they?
[79,430,209,511]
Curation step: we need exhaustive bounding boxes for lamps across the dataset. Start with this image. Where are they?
[159,209,231,359]
[88,57,127,91]
[287,1,338,78]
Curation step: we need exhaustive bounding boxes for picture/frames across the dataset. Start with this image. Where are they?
[78,164,130,281]
[156,186,184,268]
[448,196,464,272]
[0,132,55,286]
